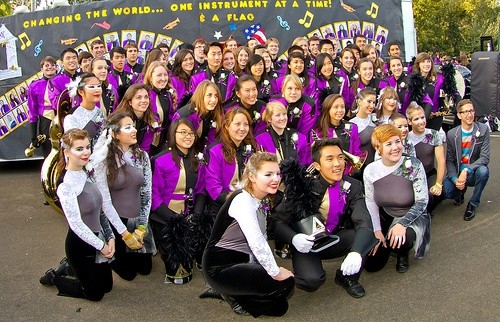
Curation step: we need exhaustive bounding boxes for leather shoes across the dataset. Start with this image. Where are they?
[464,201,476,221]
[198,288,226,301]
[54,257,70,277]
[220,291,250,316]
[454,184,467,206]
[40,268,58,287]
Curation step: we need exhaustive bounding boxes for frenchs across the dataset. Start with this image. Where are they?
[40,86,72,216]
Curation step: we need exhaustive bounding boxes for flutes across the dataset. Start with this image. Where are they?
[106,84,112,106]
[106,93,114,139]
[188,188,193,217]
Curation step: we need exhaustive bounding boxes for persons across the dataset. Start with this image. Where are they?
[47,35,500,167]
[442,99,491,221]
[150,118,206,286]
[310,24,386,57]
[90,110,157,281]
[308,94,362,177]
[78,33,181,73]
[203,107,270,219]
[402,101,446,218]
[199,151,295,319]
[363,124,431,273]
[254,100,308,206]
[27,56,59,205]
[40,128,115,302]
[270,137,374,298]
[0,64,60,138]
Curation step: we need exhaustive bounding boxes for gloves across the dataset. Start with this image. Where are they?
[133,224,147,244]
[122,233,142,250]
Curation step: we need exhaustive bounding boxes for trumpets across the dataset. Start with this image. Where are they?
[311,129,368,172]
[429,89,457,124]
[24,134,46,158]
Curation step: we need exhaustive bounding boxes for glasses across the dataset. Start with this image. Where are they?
[459,110,475,114]
[176,131,197,136]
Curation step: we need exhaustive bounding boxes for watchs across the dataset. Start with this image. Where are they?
[463,167,469,172]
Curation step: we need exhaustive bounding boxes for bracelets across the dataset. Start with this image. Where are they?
[436,181,443,187]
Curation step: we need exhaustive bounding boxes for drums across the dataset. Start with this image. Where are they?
[453,66,471,99]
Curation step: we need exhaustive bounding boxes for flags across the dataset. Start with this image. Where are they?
[244,23,268,46]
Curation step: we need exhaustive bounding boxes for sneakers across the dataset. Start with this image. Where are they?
[335,269,365,299]
[396,254,408,273]
